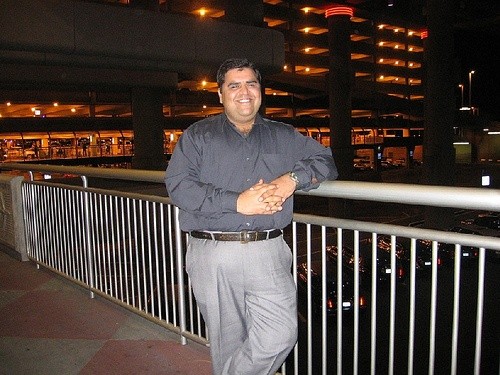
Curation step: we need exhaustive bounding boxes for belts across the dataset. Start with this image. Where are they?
[188,228,282,243]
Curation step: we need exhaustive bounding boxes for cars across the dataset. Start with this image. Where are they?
[474,211,500,231]
[325,238,409,288]
[353,159,406,172]
[364,226,490,276]
[295,259,370,321]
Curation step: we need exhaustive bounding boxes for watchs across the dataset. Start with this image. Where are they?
[288,171,300,190]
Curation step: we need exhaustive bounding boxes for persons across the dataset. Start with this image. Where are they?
[33,143,40,159]
[80,141,87,157]
[99,139,111,155]
[163,58,338,375]
